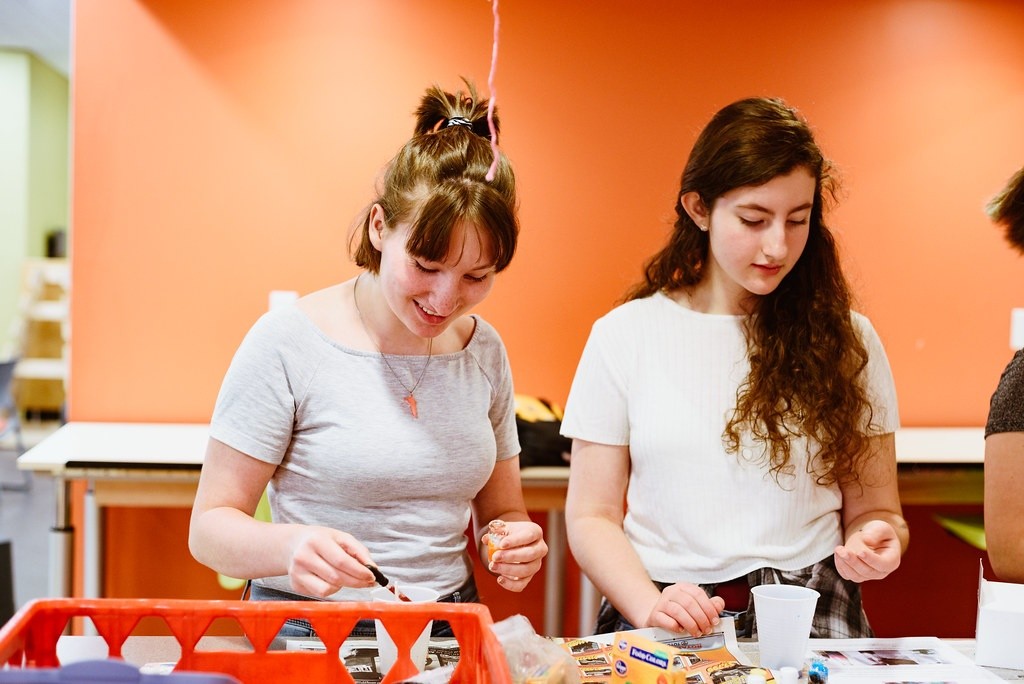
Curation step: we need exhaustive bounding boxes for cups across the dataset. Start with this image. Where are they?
[378,586,442,680]
[750,584,820,672]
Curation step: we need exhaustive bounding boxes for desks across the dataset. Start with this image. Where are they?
[5,637,1024,684]
[16,419,579,636]
[581,426,987,637]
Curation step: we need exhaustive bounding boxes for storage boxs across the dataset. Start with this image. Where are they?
[0,597,514,684]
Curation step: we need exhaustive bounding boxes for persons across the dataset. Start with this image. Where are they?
[558,96,909,639]
[983,170,1024,583]
[188,78,549,639]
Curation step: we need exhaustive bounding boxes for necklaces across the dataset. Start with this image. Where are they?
[354,274,432,418]
